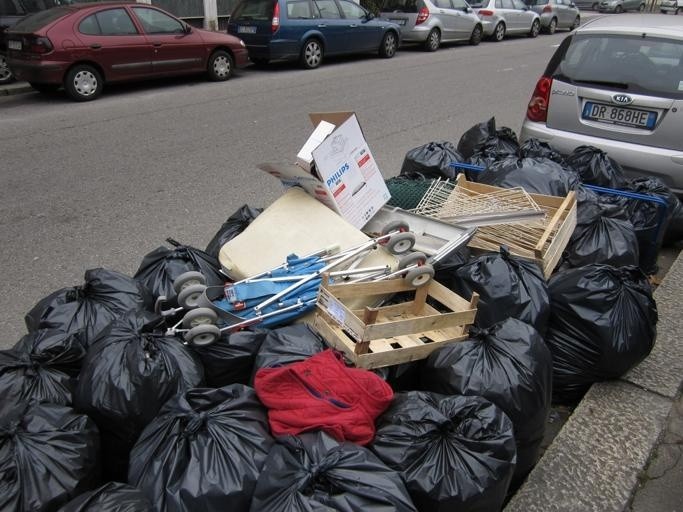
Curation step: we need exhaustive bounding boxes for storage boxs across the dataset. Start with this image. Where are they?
[257,108,391,229]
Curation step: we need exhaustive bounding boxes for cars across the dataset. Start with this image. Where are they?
[517,11,682,202]
[570,0,682,15]
[374,0,483,50]
[223,0,404,72]
[0,14,31,88]
[523,0,581,35]
[464,0,542,42]
[0,2,76,85]
[0,0,251,103]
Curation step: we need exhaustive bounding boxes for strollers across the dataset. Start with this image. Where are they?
[151,216,437,350]
